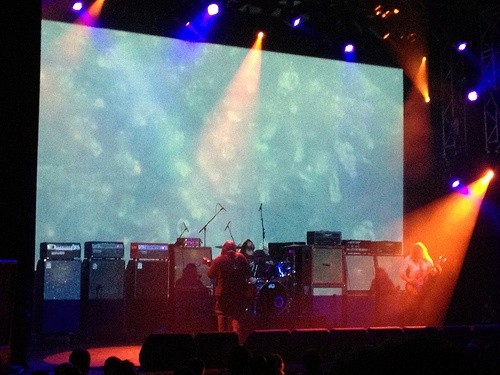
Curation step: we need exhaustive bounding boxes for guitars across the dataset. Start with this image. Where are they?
[406,259,448,298]
[201,257,257,304]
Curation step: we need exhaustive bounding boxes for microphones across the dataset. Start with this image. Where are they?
[183,223,188,232]
[225,221,230,230]
[258,204,262,211]
[218,203,227,211]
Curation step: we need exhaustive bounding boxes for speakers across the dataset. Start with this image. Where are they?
[301,246,343,287]
[341,252,375,292]
[172,247,214,301]
[81,260,126,300]
[125,260,172,302]
[37,259,82,300]
[374,255,406,289]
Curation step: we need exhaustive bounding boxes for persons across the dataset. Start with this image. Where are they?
[54,333,482,375]
[398,240,439,325]
[239,239,255,264]
[207,240,249,332]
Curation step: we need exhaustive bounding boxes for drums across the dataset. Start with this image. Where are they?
[254,250,274,274]
[247,279,289,320]
[275,260,295,286]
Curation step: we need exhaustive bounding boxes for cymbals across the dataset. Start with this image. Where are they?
[214,246,247,248]
[282,243,308,248]
